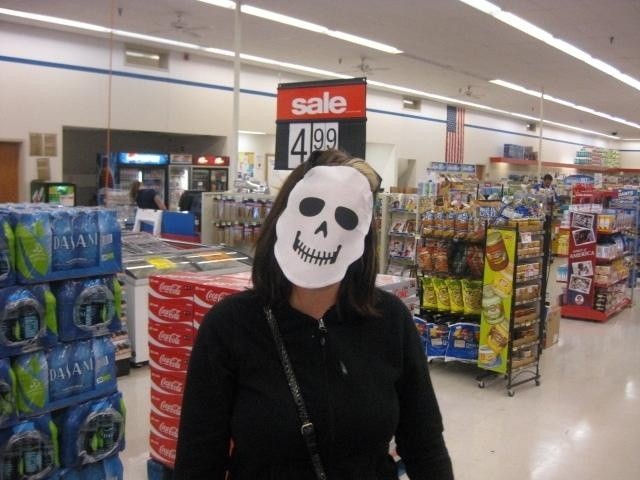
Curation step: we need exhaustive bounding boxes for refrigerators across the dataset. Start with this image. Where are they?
[118,230,259,368]
[95,151,168,230]
[192,154,231,235]
[169,153,192,211]
[30,180,76,206]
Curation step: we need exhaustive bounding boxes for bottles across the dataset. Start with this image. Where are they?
[416,179,437,198]
[1,203,125,480]
[97,154,114,204]
[119,166,140,193]
[210,195,277,247]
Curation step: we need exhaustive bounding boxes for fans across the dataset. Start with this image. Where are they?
[144,10,213,41]
[334,53,393,74]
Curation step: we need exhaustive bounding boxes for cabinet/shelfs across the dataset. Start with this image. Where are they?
[555,190,633,323]
[200,190,417,280]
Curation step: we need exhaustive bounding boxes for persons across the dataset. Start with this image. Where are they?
[174,149,454,480]
[136,180,166,211]
[534,173,557,199]
[131,179,142,198]
